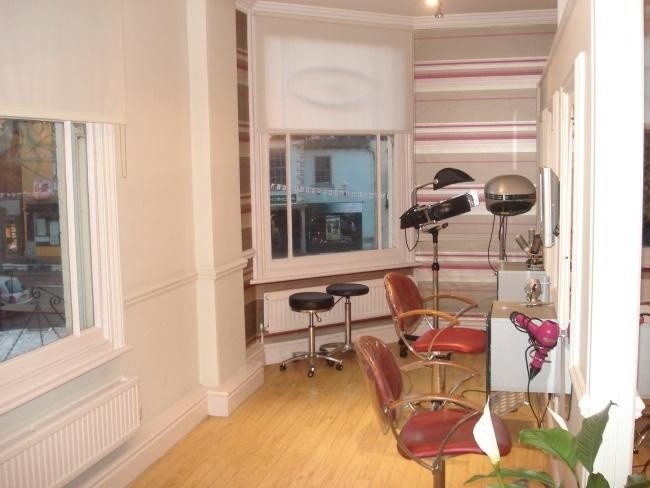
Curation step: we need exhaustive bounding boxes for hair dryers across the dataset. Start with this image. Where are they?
[512,313,559,380]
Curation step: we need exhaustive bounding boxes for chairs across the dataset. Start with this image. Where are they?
[353,272,512,487]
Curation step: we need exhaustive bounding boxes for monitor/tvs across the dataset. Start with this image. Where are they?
[270,204,306,259]
[306,202,362,255]
[539,167,559,248]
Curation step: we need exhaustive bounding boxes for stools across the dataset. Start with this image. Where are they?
[278,282,370,377]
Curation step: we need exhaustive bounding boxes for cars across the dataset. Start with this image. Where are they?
[0,276,33,318]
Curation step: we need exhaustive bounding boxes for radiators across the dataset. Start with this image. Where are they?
[265,275,419,336]
[2,374,142,486]
